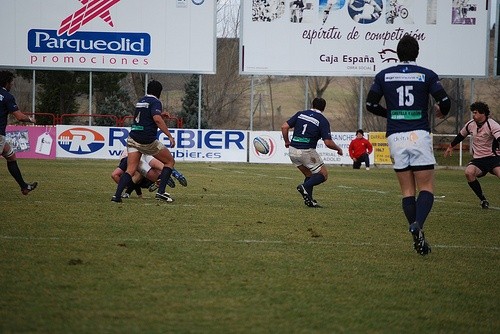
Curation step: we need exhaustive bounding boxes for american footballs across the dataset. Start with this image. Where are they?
[254,137,269,154]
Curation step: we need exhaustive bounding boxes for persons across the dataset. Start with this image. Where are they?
[281,97,343,208]
[0,70,39,196]
[365,36,452,256]
[111,80,175,202]
[443,102,500,208]
[349,129,373,171]
[110,149,187,198]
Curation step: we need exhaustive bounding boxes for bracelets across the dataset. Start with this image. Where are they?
[29,117,32,122]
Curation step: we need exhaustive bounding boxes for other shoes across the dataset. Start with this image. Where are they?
[154,192,173,203]
[21,181,38,195]
[297,184,320,206]
[366,167,370,170]
[480,200,489,209]
[408,223,431,256]
[148,179,161,192]
[110,195,123,204]
[167,169,187,188]
